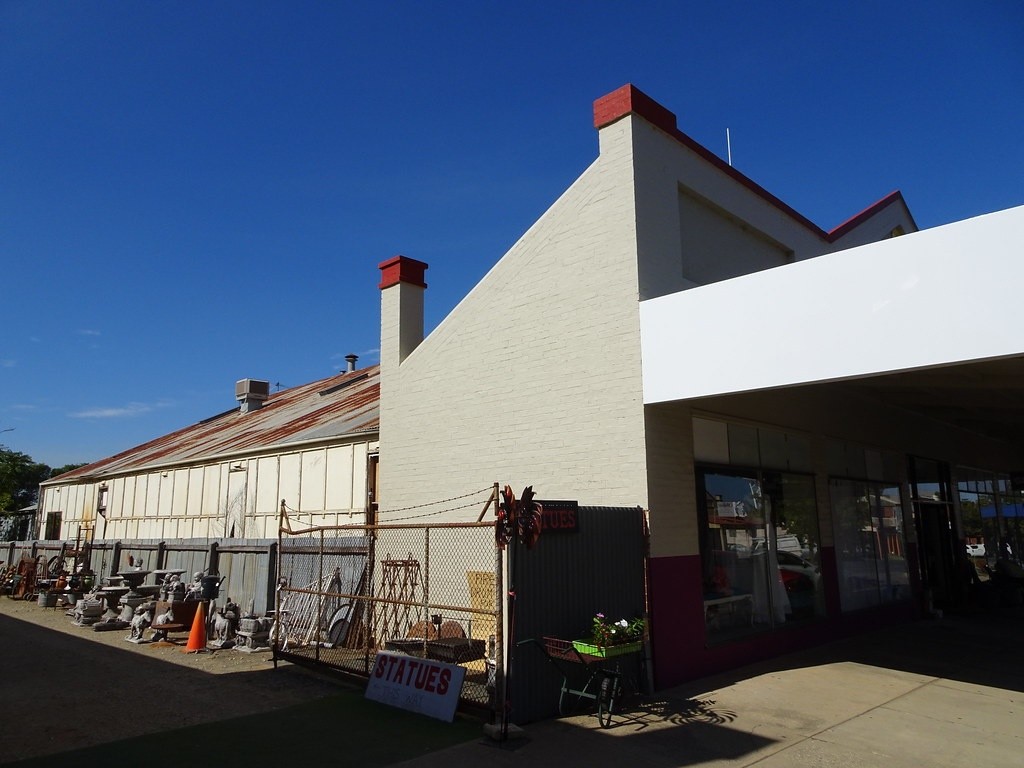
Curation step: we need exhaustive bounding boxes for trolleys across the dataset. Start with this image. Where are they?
[514,635,645,729]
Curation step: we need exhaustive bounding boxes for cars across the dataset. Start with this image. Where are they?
[708,533,1014,625]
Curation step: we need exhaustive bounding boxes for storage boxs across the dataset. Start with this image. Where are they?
[573,640,642,658]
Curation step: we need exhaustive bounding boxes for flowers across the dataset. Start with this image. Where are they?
[591,612,645,644]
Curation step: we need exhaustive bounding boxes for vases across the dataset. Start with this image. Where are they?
[600,638,639,646]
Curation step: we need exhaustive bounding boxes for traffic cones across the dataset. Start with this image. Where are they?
[178,601,208,654]
